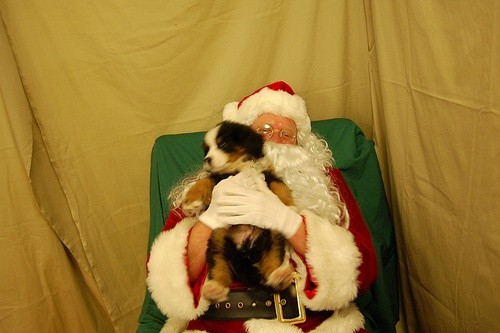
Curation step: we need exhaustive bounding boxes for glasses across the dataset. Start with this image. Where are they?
[256,123,297,144]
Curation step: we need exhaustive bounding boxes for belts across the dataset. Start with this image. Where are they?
[199,271,310,324]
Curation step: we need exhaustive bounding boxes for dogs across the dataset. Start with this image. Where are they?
[182,120,298,303]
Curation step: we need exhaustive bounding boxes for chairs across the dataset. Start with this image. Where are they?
[133,118,401,333]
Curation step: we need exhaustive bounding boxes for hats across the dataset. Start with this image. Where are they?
[222,80,312,134]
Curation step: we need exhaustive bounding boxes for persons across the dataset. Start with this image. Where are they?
[145,80,381,333]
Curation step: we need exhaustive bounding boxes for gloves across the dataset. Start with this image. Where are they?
[213,166,303,240]
[196,167,267,230]
